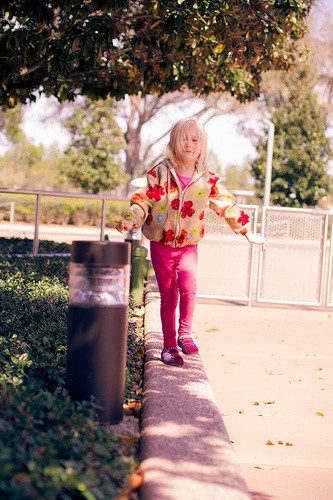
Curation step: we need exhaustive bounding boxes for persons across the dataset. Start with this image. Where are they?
[115,117,267,366]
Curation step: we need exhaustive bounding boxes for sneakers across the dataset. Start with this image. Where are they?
[177,334,199,355]
[160,347,184,366]
